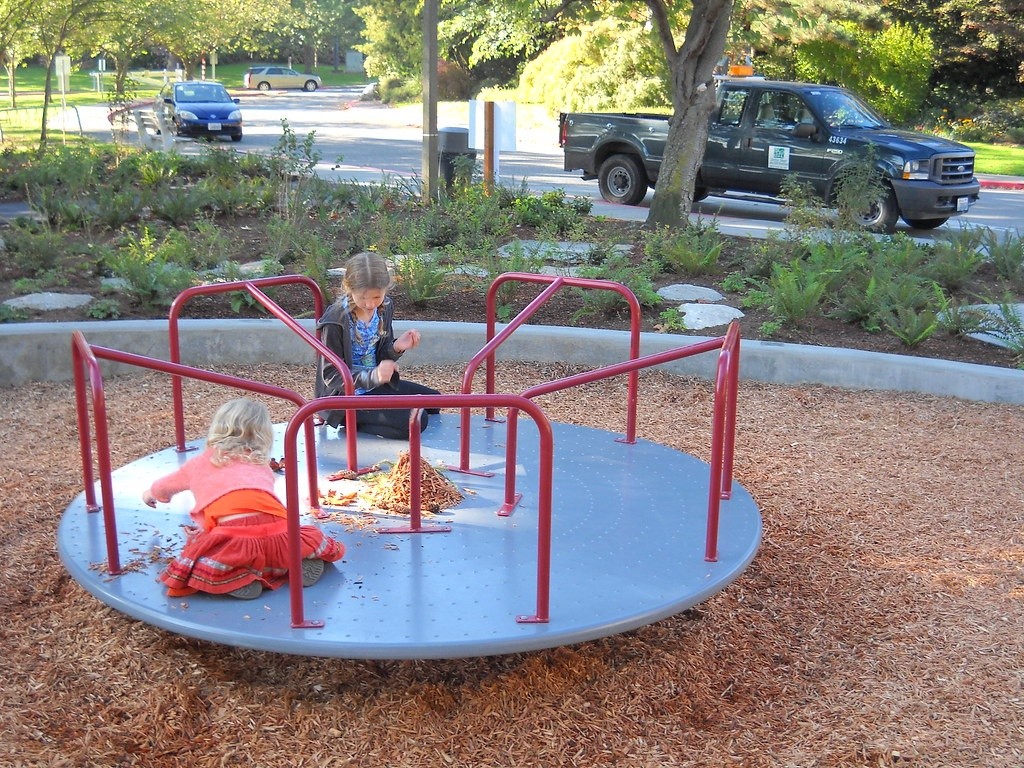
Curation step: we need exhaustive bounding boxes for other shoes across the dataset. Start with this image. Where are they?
[229,554,324,599]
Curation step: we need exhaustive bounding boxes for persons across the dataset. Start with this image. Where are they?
[314,251,442,439]
[140,395,325,600]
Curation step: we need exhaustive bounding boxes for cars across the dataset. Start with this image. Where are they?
[154,81,243,143]
[243,65,324,92]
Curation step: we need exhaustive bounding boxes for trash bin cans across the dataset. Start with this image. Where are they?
[437,127,476,197]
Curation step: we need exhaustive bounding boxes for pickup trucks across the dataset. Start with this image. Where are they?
[557,76,979,235]
